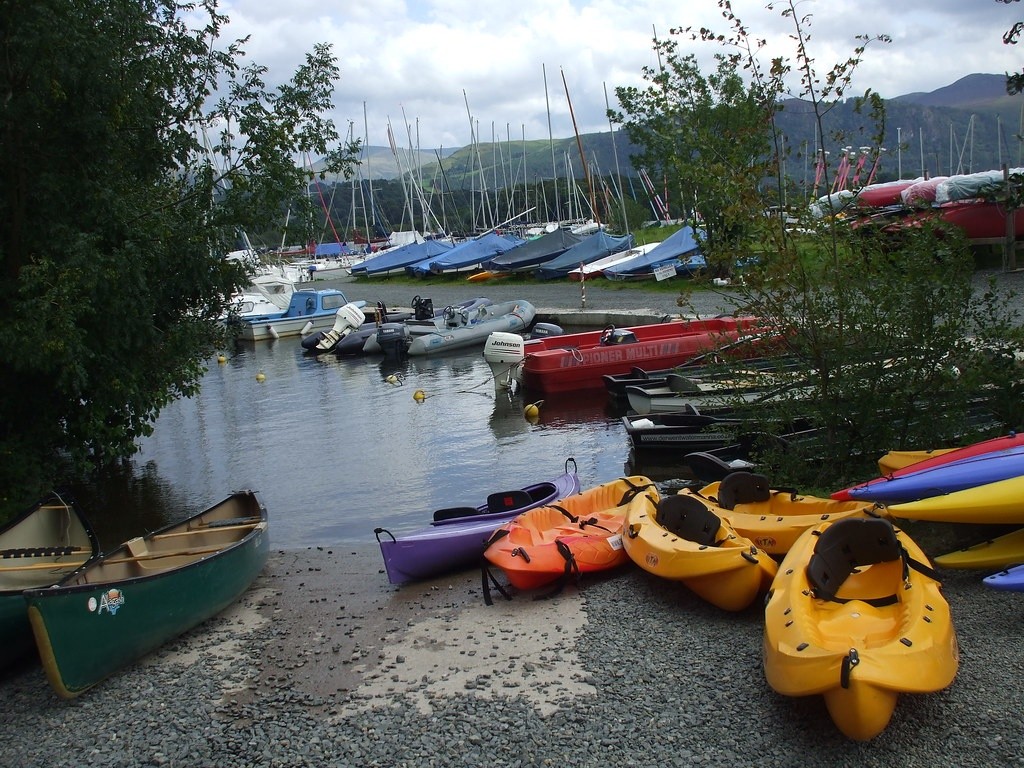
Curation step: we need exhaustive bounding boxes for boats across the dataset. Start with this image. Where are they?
[622,480,778,611]
[185,278,537,358]
[478,315,1024,479]
[813,163,1023,244]
[828,430,1024,590]
[693,471,889,555]
[373,456,579,588]
[760,515,960,743]
[480,474,660,594]
[0,485,272,700]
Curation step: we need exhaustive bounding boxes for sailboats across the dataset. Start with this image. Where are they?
[202,24,776,284]
